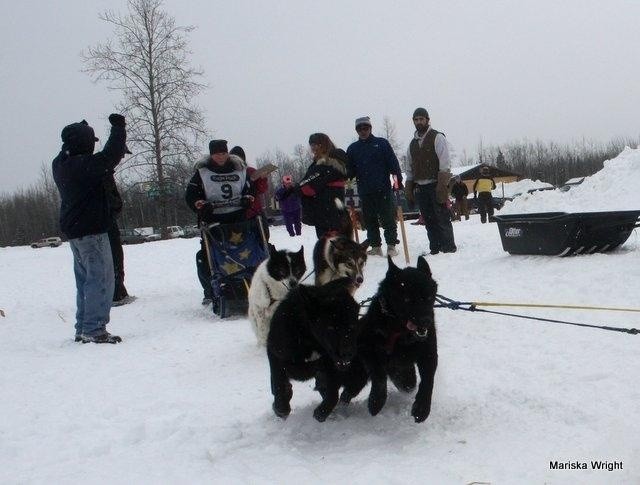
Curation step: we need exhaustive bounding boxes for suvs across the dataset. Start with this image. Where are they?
[29,236,62,249]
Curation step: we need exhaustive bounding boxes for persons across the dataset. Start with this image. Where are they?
[297,133,354,242]
[229,146,269,244]
[473,166,497,222]
[52,113,126,344]
[107,171,128,308]
[405,108,457,254]
[344,117,403,258]
[451,174,469,221]
[185,140,255,306]
[274,176,302,236]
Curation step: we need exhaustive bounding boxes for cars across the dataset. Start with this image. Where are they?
[116,222,204,246]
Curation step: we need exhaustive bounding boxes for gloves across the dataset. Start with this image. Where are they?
[107,114,127,131]
[404,180,416,211]
[434,170,453,203]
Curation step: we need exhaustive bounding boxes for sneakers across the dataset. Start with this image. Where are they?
[75,332,123,346]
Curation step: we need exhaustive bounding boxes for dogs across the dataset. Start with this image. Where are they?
[338,254,439,424]
[246,241,308,351]
[312,233,372,297]
[265,275,363,422]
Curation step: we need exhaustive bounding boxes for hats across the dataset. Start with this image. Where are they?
[412,107,431,121]
[354,115,373,129]
[228,145,247,164]
[60,122,99,145]
[208,138,229,157]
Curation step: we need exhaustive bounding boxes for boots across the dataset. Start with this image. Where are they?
[387,244,398,258]
[367,246,384,258]
